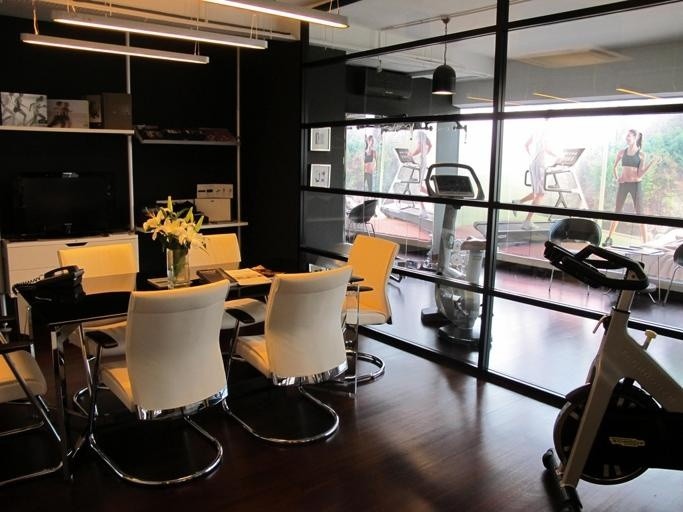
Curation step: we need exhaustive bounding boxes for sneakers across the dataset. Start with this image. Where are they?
[601,237,611,246]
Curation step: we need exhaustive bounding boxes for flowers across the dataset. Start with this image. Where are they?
[127,194,213,285]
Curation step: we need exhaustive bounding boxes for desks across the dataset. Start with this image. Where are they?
[601,239,674,305]
[17,261,366,439]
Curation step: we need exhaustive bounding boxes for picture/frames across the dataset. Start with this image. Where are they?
[308,125,332,153]
[308,163,331,190]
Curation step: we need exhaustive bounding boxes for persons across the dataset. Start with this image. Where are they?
[1,92,73,129]
[511,116,559,230]
[364,135,377,217]
[602,129,659,247]
[407,128,432,212]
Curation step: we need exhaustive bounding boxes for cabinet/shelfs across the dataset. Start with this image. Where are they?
[0,1,249,247]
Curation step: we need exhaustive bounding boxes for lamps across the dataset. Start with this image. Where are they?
[430,16,458,97]
[16,1,352,66]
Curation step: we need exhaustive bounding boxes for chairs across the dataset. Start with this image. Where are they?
[542,216,604,299]
[74,277,230,485]
[221,266,352,444]
[1,347,64,487]
[344,198,377,244]
[57,243,138,278]
[661,243,683,305]
[309,235,400,384]
[167,234,242,268]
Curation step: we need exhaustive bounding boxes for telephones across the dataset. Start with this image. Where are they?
[34,265,85,291]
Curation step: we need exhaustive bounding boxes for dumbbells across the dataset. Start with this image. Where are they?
[648,153,663,164]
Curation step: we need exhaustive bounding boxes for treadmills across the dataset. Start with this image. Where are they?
[473,147,589,247]
[380,147,434,234]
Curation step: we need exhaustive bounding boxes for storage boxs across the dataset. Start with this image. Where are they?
[195,183,235,199]
[154,195,232,223]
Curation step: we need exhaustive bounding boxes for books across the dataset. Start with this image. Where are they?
[196,263,276,288]
